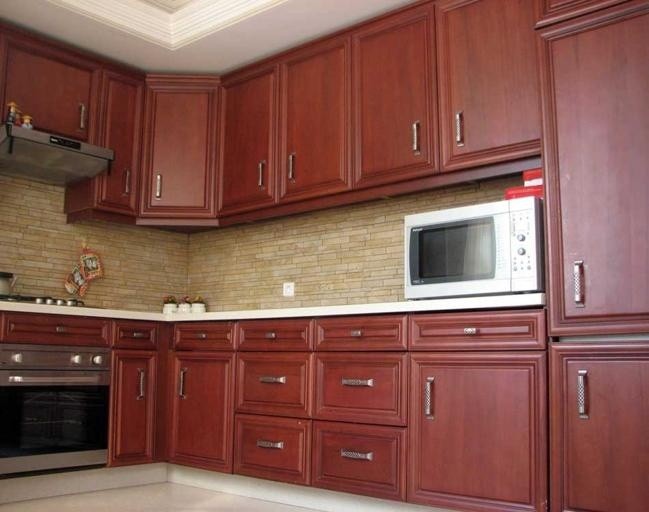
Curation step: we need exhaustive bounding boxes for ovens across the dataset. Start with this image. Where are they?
[0,371,111,477]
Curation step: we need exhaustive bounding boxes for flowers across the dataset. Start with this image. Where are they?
[178,295,192,304]
[193,296,205,304]
[163,294,177,304]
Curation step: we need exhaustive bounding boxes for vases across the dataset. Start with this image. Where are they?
[164,304,177,313]
[192,304,206,312]
[179,304,191,313]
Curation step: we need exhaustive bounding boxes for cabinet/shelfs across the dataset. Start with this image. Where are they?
[223,27,352,224]
[312,316,406,501]
[532,0,649,512]
[0,309,111,349]
[407,311,544,512]
[1,27,103,144]
[111,317,162,467]
[164,322,233,475]
[354,0,544,203]
[64,58,221,231]
[235,316,311,486]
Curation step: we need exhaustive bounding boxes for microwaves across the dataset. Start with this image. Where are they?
[402,197,544,302]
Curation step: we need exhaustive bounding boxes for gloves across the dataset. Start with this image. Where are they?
[65,265,89,297]
[77,247,103,282]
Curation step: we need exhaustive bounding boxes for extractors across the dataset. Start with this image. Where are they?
[0,124,116,191]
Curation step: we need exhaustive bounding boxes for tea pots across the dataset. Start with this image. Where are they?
[0,272,19,296]
